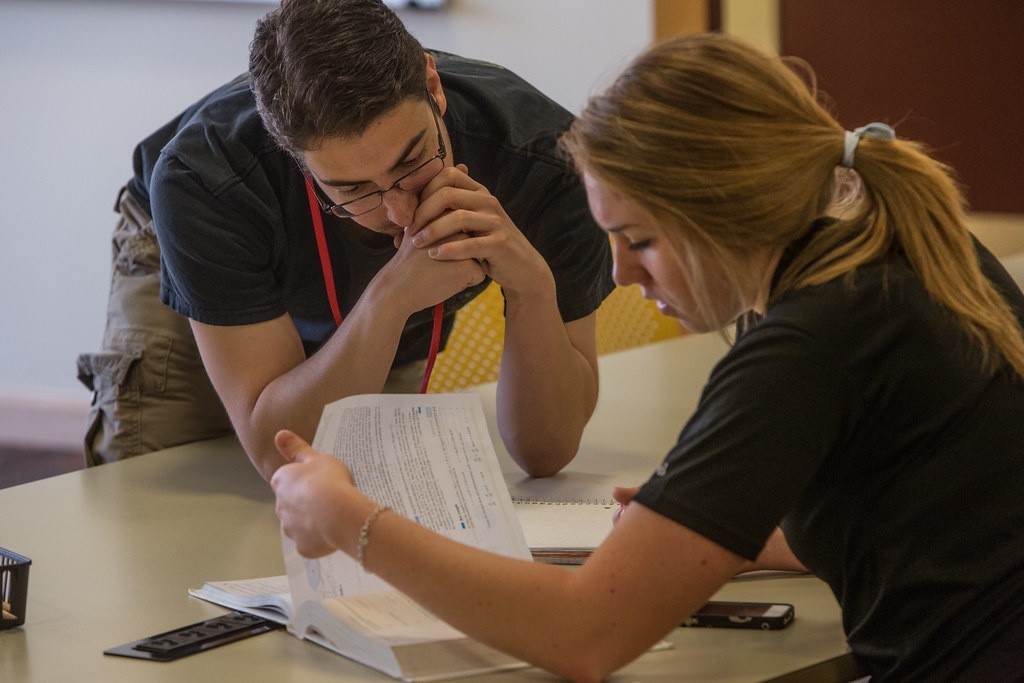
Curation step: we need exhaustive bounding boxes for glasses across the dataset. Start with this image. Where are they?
[287,85,448,219]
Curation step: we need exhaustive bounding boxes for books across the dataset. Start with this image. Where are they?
[187,390,619,683]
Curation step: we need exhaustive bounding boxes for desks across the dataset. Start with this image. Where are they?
[0,256,1024,683]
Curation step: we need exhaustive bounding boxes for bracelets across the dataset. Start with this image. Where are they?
[357,505,391,571]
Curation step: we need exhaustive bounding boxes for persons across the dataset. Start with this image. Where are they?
[76,0,616,484]
[266,33,1024,683]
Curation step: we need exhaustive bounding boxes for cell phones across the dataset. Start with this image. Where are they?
[681,602,794,628]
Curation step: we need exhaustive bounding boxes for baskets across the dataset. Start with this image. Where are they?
[0,547,32,631]
